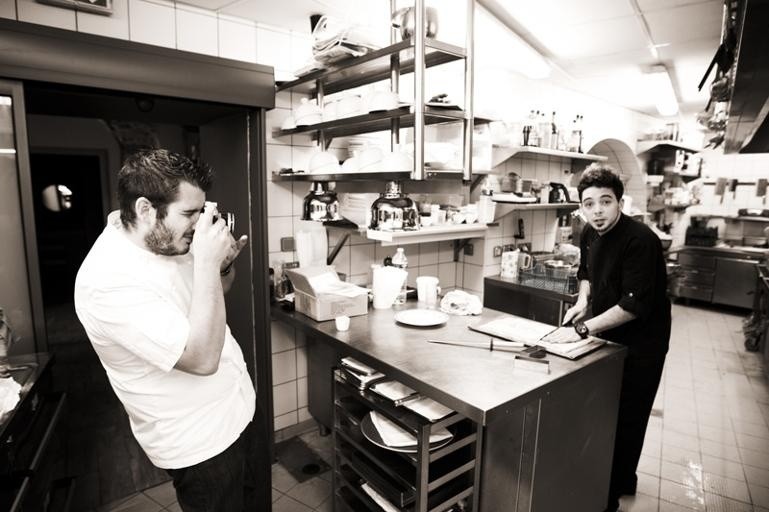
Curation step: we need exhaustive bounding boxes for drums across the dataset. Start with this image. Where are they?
[714,177,727,204]
[539,320,574,340]
[756,178,768,204]
[429,338,530,352]
[728,179,738,200]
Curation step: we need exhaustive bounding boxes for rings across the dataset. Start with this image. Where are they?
[515,356,551,374]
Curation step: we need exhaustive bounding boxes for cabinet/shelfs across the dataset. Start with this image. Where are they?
[391,248,407,306]
[522,110,583,153]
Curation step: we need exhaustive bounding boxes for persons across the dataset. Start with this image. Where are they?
[73,149,271,511]
[562,162,673,512]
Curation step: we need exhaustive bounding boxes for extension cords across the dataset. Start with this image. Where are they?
[299,180,346,224]
[368,182,421,231]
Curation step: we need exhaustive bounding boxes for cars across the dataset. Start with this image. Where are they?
[416,276,441,303]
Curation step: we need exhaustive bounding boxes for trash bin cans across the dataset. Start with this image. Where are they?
[577,324,591,338]
[215,264,235,276]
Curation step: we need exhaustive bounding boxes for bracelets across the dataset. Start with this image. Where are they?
[298,147,341,174]
[282,87,400,130]
[544,259,573,277]
[341,143,462,174]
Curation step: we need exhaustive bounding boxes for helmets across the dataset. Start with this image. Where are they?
[395,308,450,326]
[347,138,370,158]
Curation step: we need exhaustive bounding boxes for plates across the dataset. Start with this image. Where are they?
[330,369,481,512]
[273,288,629,512]
[0,352,69,512]
[674,248,765,311]
[484,276,591,325]
[270,0,475,184]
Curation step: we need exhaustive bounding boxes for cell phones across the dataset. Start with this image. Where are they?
[205,201,235,233]
